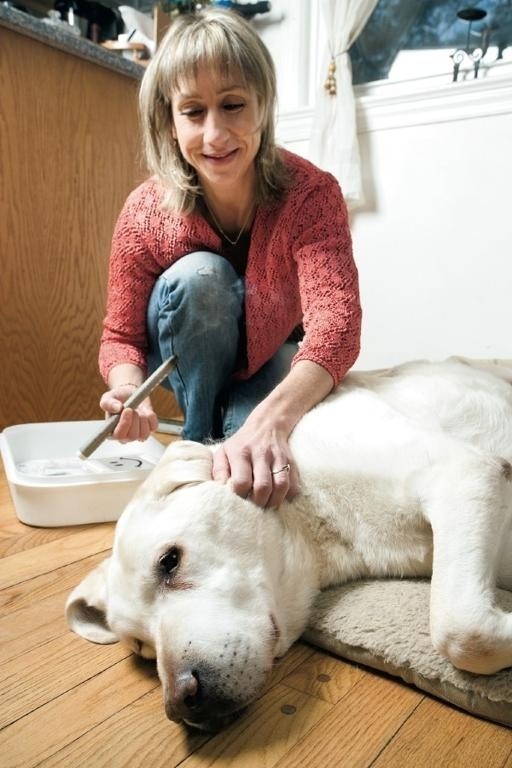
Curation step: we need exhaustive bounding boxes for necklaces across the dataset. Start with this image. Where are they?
[204,195,257,245]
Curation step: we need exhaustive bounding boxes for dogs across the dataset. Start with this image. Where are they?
[62,350,512,738]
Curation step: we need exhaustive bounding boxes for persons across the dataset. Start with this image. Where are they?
[97,4,365,513]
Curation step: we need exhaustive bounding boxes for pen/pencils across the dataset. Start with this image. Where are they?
[126,29,135,41]
[78,352,178,462]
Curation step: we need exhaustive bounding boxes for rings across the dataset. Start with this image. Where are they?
[271,464,292,477]
